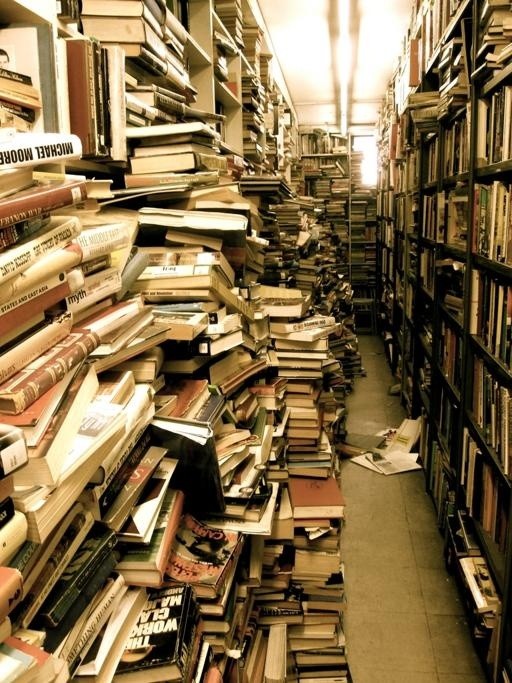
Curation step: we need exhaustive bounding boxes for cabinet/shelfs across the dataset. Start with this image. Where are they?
[0,0,299,203]
[376,0,512,683]
[299,131,376,287]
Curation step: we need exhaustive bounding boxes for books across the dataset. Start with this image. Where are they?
[0,0,511,683]
